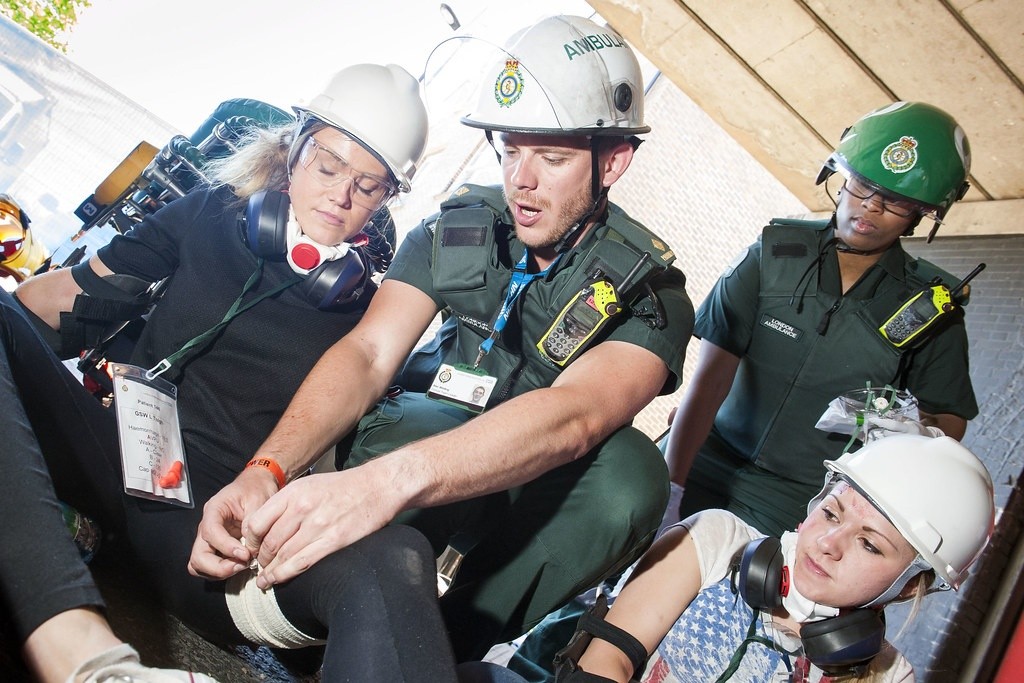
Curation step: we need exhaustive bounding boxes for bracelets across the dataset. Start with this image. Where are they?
[246,459,286,489]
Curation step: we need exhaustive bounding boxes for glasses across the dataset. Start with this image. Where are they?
[844,174,926,218]
[299,135,400,212]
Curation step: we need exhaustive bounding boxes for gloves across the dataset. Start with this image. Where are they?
[866,417,945,442]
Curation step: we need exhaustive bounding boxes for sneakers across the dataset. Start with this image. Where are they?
[68,643,221,683]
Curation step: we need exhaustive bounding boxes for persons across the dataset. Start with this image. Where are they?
[0,13,996,683]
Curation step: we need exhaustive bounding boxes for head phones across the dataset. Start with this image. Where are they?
[731,537,887,676]
[241,188,370,313]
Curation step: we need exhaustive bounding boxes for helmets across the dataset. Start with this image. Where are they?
[815,101,971,243]
[285,64,429,194]
[790,434,995,614]
[460,14,651,253]
